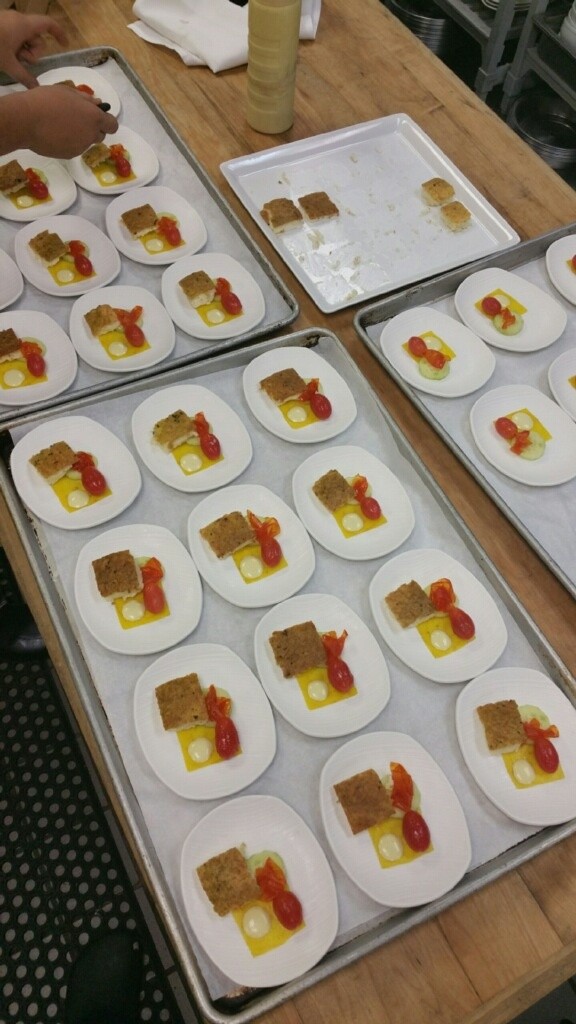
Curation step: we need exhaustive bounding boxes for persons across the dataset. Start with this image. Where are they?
[64,928,144,1024]
[0,9,119,661]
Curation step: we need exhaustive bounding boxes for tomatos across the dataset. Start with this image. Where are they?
[20,154,559,931]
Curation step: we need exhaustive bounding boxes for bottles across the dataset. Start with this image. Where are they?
[245,0,302,135]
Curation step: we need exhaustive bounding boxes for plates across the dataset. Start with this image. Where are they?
[74,524,203,655]
[0,66,266,405]
[179,794,340,987]
[380,306,497,398]
[291,446,415,561]
[546,233,576,422]
[132,384,255,492]
[469,384,575,486]
[10,417,142,530]
[186,485,316,607]
[133,643,277,802]
[243,345,359,443]
[255,593,392,738]
[368,548,509,685]
[318,731,473,907]
[455,266,567,352]
[456,667,575,826]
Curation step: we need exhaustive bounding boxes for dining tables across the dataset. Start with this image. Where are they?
[0,0,576,1024]
